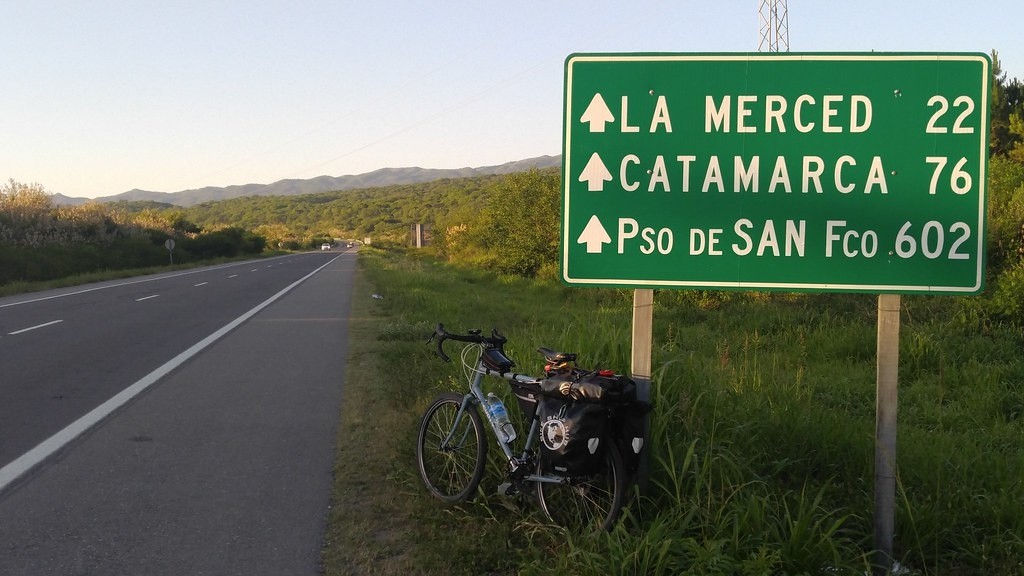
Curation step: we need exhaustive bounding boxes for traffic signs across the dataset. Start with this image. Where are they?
[560,50,993,296]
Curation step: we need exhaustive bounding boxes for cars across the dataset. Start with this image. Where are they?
[321,243,331,252]
[347,244,353,248]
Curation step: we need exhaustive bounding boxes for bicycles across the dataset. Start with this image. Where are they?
[414,322,625,543]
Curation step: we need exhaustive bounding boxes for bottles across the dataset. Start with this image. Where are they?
[487,392,516,443]
[372,294,384,300]
